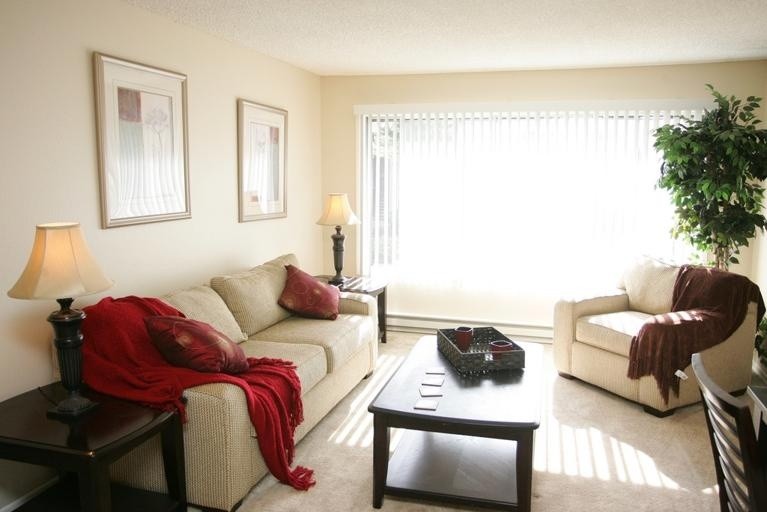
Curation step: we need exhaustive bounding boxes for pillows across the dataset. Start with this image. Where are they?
[277,264,340,319]
[143,315,251,372]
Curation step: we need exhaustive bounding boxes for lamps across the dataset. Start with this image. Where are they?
[6,220,114,419]
[317,193,360,284]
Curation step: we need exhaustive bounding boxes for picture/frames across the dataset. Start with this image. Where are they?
[92,51,192,230]
[237,97,288,222]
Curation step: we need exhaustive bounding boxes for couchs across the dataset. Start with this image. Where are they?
[552,258,757,417]
[53,254,378,512]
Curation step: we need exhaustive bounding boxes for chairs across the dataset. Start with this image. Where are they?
[691,353,767,511]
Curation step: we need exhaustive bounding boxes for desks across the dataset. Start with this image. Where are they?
[313,276,388,342]
[0,383,186,512]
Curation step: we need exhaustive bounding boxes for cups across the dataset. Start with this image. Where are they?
[490,340,513,360]
[455,326,473,350]
